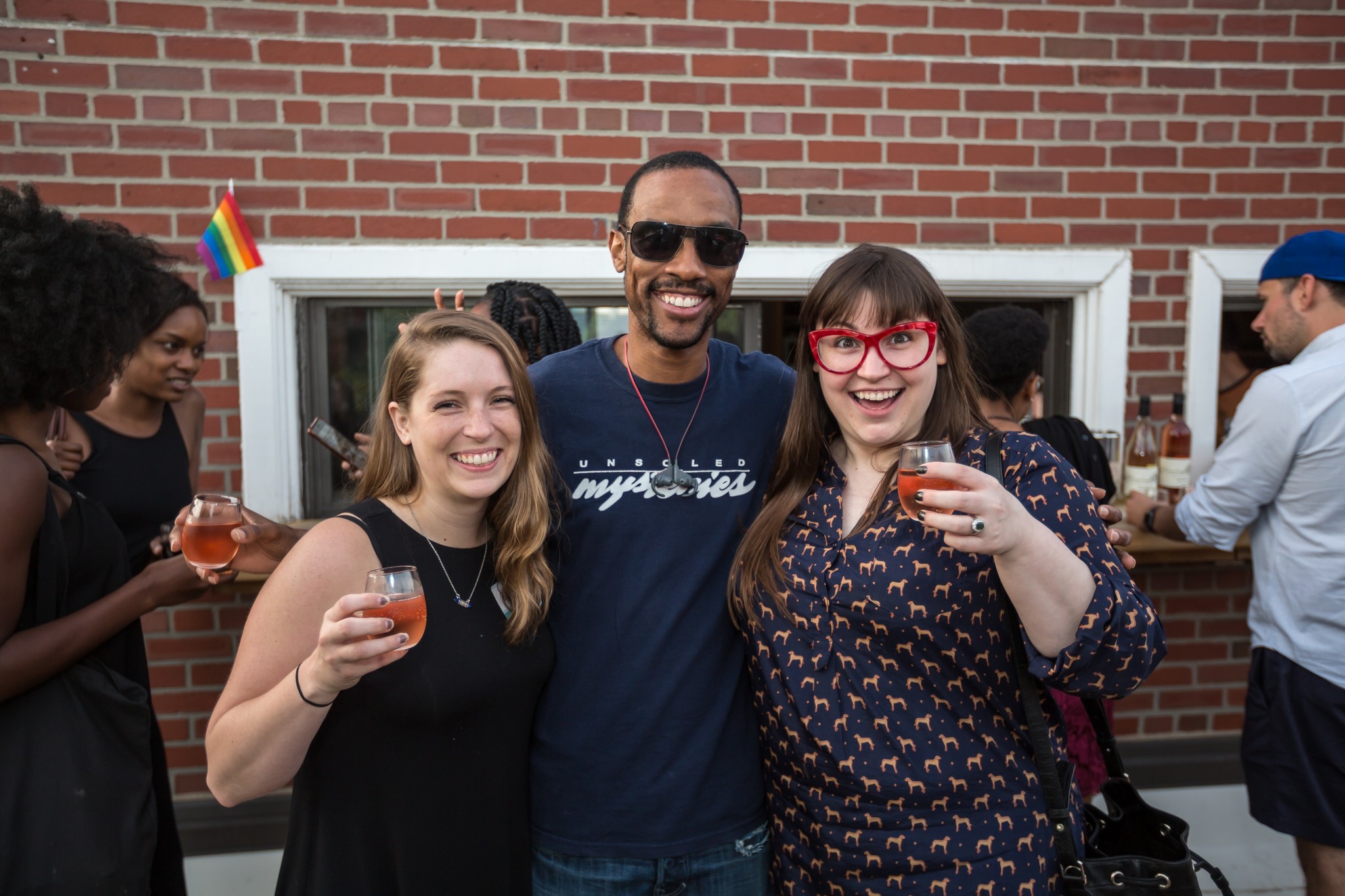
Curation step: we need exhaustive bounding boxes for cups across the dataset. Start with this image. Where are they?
[897,439,957,521]
[362,564,427,651]
[182,493,243,568]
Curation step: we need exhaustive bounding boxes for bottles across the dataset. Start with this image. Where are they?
[1123,395,1159,504]
[1159,393,1192,504]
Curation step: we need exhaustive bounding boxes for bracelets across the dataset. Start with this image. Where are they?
[1143,504,1162,534]
[294,658,339,711]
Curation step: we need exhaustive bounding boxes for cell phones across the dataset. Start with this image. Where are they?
[306,416,369,471]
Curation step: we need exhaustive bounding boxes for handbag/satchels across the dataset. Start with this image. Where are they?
[1060,779,1234,896]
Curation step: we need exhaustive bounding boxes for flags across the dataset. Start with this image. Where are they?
[196,188,265,281]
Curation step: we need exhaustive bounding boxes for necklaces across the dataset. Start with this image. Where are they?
[400,489,491,611]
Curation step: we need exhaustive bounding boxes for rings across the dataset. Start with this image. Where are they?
[970,515,987,539]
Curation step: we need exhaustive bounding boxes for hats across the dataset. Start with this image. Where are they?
[1258,229,1345,282]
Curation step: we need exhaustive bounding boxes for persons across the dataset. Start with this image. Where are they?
[339,279,583,486]
[201,310,558,896]
[1128,224,1344,896]
[0,184,240,896]
[151,150,1138,896]
[725,237,1171,896]
[953,301,1116,803]
[46,268,204,579]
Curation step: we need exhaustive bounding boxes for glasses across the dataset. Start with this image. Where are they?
[618,222,749,267]
[808,321,938,375]
